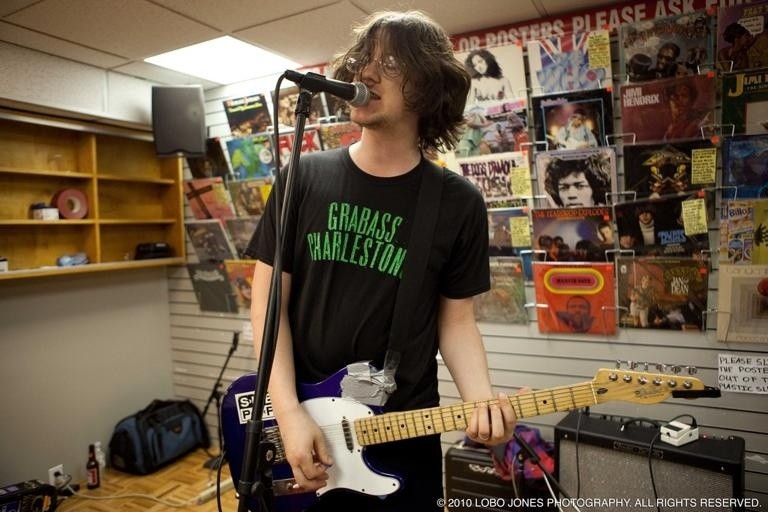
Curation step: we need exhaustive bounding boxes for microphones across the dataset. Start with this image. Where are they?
[284,69,371,108]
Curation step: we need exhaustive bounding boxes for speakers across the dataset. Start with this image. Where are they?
[151,84,208,157]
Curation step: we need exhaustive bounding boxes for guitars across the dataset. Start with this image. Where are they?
[220,360,721,512]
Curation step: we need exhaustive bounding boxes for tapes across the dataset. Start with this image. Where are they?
[32,188,89,221]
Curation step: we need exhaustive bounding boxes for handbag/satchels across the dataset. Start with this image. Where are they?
[108,399,211,476]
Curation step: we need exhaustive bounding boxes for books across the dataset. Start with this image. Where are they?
[183,86,532,327]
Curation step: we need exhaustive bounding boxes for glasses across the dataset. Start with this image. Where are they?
[344,52,404,77]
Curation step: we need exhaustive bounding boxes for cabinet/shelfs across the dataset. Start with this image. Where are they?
[0,107,186,279]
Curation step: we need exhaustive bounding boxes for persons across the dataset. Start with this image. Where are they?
[243,11,518,512]
[537,16,768,332]
[456,49,517,156]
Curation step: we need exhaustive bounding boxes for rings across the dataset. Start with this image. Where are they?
[478,434,490,441]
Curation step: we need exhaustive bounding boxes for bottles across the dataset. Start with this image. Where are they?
[88,441,105,490]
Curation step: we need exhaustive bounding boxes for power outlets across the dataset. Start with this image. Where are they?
[48,464,64,486]
[243,322,253,341]
